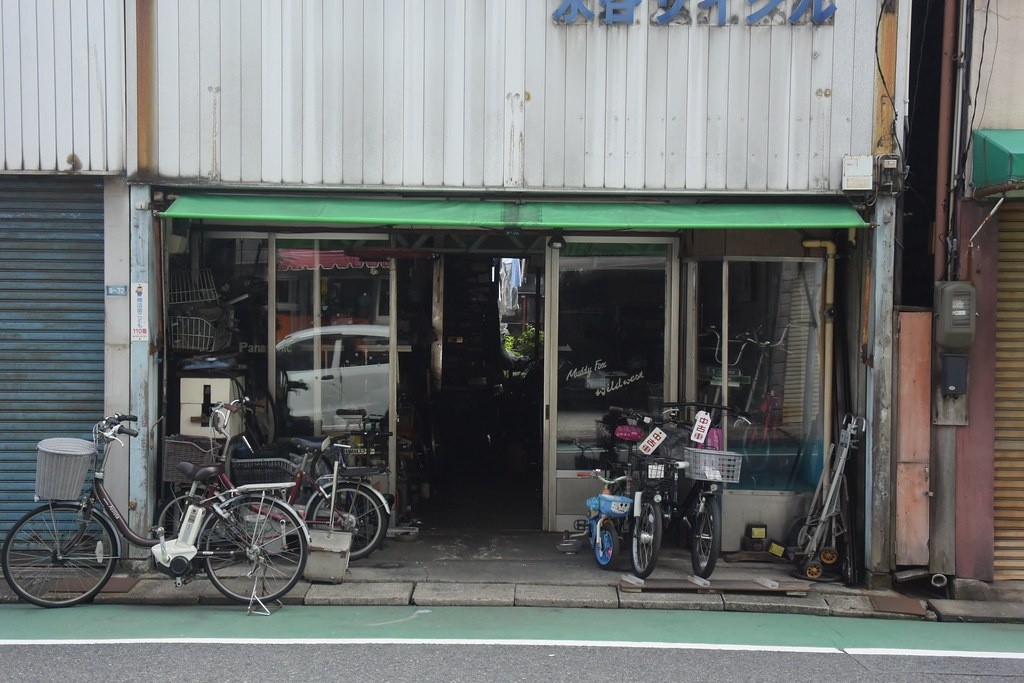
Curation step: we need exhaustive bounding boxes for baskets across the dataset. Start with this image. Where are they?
[599,494,634,518]
[631,452,680,492]
[162,434,221,483]
[683,446,742,484]
[35,436,96,501]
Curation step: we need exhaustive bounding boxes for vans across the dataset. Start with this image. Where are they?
[273,325,392,435]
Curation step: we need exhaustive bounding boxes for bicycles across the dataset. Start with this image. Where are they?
[0,414,312,617]
[561,402,754,579]
[157,395,392,562]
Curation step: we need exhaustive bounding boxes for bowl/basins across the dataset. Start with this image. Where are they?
[395,532,418,542]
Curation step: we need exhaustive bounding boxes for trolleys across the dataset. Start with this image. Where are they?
[793,412,867,582]
